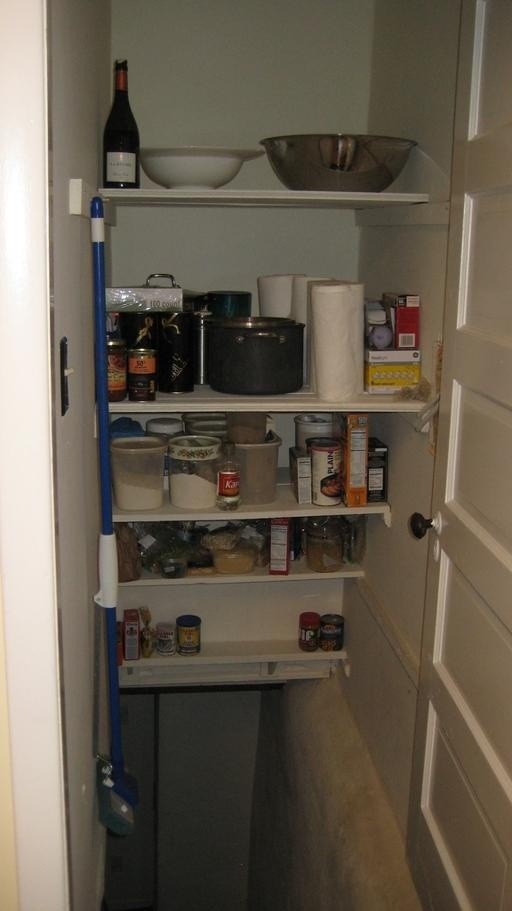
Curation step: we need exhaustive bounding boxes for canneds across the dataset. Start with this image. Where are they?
[107,339,127,402]
[320,614,344,651]
[127,349,157,402]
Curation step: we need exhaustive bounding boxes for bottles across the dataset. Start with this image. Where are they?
[100,59,141,189]
[304,519,343,573]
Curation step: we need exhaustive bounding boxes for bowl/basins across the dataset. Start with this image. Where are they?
[210,543,257,574]
[138,133,417,193]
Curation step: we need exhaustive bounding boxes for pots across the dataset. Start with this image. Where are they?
[204,315,305,396]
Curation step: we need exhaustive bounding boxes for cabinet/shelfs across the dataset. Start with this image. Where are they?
[93,185,430,692]
